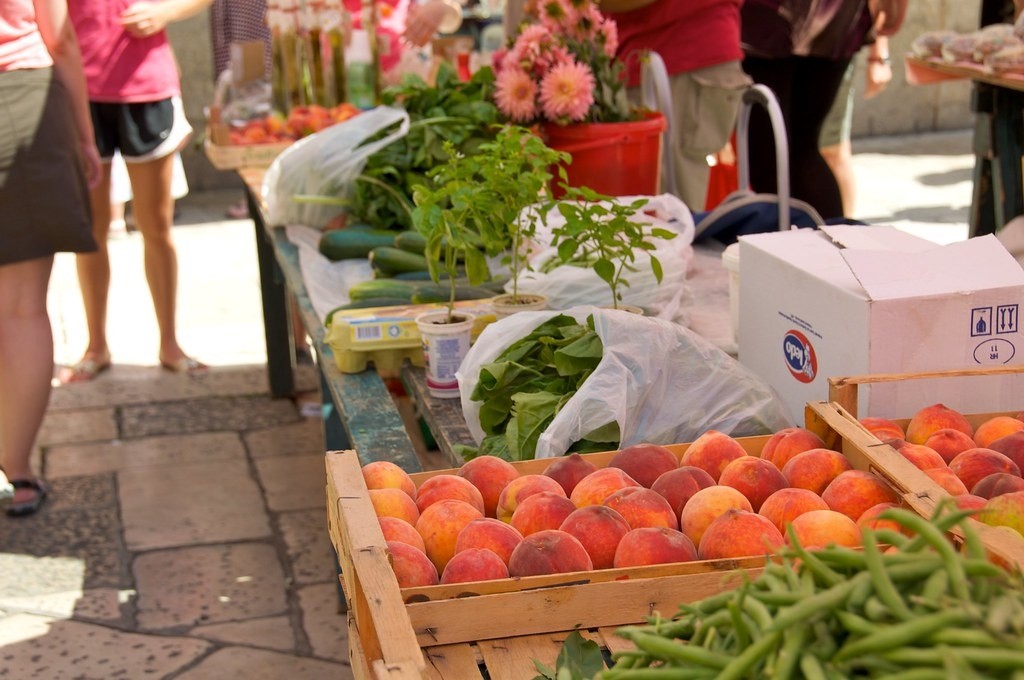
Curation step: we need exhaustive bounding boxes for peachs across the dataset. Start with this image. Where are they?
[361,425,909,589]
[228,103,360,147]
[856,400,1024,550]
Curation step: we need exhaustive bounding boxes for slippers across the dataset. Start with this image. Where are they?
[161,358,208,377]
[71,361,110,380]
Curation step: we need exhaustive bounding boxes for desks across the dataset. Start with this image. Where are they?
[903,58,1024,239]
[237,167,477,616]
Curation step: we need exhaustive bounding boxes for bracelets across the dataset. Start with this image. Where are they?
[869,55,891,64]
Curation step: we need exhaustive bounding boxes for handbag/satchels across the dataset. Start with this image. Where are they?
[260,107,410,228]
[503,192,696,329]
[454,305,797,460]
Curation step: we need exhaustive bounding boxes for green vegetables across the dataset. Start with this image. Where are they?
[450,313,621,462]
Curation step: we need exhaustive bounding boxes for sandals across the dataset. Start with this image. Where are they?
[6,478,49,516]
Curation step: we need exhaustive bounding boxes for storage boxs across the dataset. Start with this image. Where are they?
[325,223,1024,680]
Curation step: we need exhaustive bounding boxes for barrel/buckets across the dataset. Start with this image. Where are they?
[538,109,667,200]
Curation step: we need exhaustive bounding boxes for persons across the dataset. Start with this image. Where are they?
[597,0,754,214]
[68,0,215,383]
[740,0,893,220]
[0,0,103,518]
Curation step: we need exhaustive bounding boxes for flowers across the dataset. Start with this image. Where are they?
[479,0,644,126]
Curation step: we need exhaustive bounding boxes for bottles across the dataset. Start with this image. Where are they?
[209,1,505,146]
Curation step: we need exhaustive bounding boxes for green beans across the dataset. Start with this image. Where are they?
[592,495,1024,680]
